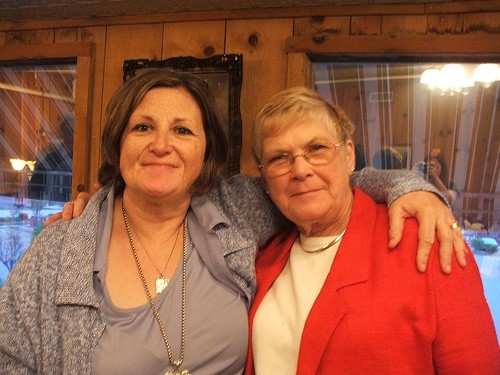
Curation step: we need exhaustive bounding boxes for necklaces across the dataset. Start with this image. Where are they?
[121,198,193,375]
[298,229,347,253]
[127,216,182,296]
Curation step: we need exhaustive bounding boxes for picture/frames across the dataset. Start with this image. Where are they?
[123,52,243,179]
[388,143,413,172]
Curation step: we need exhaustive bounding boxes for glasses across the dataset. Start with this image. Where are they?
[257,141,344,177]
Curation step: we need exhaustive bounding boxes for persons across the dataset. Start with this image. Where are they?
[413,147,460,209]
[42,85,500,375]
[0,70,470,375]
[374,145,403,172]
[352,145,368,171]
[27,117,72,202]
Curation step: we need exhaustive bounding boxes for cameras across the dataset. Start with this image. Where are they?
[418,162,429,173]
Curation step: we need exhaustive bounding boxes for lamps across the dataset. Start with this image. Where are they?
[9,158,37,189]
[418,62,500,96]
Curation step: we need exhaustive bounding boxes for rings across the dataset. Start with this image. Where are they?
[449,222,461,230]
[63,201,74,206]
